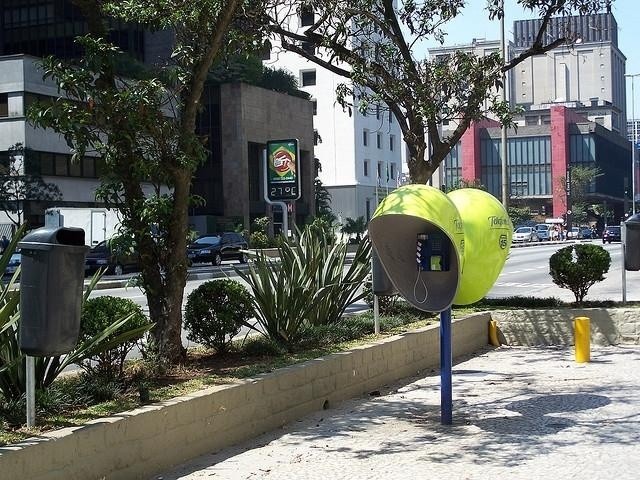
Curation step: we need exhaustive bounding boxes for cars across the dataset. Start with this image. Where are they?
[602,225,621,244]
[512,224,592,244]
[84,236,142,276]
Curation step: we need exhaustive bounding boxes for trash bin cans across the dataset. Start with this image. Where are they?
[621,213,640,307]
[21,226,90,429]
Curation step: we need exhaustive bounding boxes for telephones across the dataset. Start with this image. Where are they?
[416,234,450,272]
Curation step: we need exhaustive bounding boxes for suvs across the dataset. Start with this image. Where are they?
[186,231,249,267]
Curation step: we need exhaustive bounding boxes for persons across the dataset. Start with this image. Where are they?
[0,235,11,255]
[547,222,565,241]
[591,225,598,239]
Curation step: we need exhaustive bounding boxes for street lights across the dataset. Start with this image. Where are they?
[623,73,640,215]
